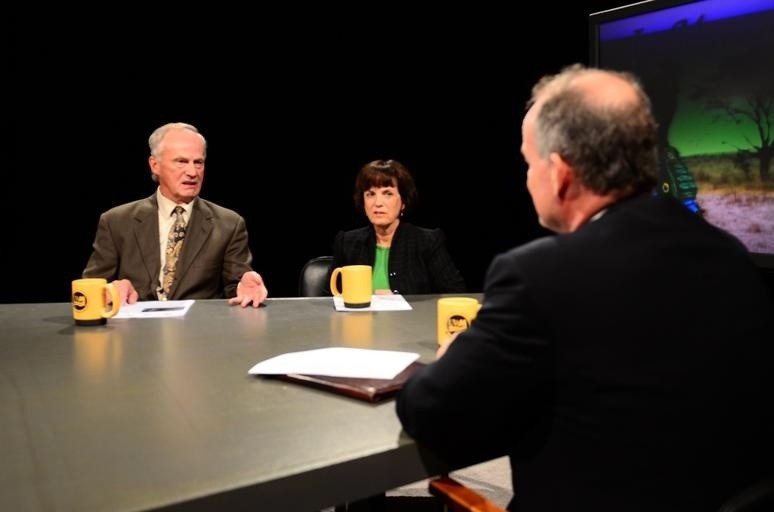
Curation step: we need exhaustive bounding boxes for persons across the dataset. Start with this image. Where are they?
[400,65,772,512]
[321,160,466,295]
[82,122,267,309]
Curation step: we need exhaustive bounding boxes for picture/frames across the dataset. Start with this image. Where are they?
[586,0,774,278]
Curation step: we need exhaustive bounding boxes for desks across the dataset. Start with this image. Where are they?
[1,287,512,512]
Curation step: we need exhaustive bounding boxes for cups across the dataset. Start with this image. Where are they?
[71,277,119,326]
[438,296,483,350]
[329,265,372,309]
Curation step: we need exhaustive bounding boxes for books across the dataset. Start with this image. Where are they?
[286,360,428,403]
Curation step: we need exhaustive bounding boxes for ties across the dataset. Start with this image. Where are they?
[163,206,187,297]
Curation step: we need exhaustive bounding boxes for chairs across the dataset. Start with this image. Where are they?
[426,470,774,512]
[300,254,338,298]
